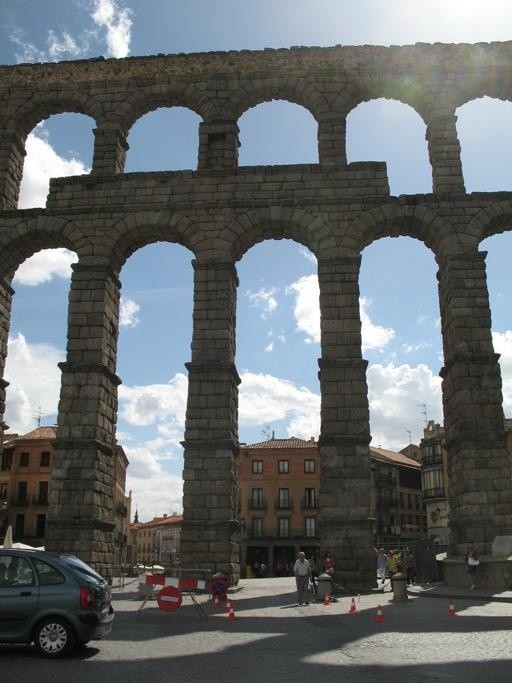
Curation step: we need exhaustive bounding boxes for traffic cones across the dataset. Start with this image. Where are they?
[324,592,330,605]
[448,600,455,616]
[375,605,385,622]
[212,592,220,609]
[226,598,237,620]
[350,598,357,613]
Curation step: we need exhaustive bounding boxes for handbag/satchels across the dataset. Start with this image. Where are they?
[468,559,480,566]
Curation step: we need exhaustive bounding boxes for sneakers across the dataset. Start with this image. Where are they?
[299,602,309,605]
[382,579,385,583]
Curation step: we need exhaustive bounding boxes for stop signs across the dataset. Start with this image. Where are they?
[156,586,182,611]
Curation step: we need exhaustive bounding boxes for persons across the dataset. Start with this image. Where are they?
[374,547,417,592]
[251,551,317,607]
[463,547,480,591]
[323,550,335,582]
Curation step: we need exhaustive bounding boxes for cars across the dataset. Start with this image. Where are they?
[0,546,116,656]
